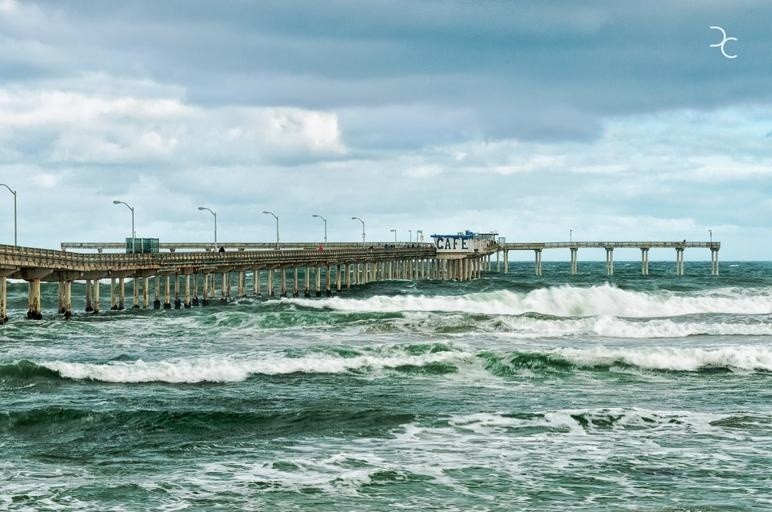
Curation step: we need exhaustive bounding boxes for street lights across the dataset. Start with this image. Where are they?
[0,183,17,247]
[198,206,217,253]
[262,210,279,250]
[312,214,327,249]
[390,229,396,242]
[113,200,134,255]
[352,217,365,250]
